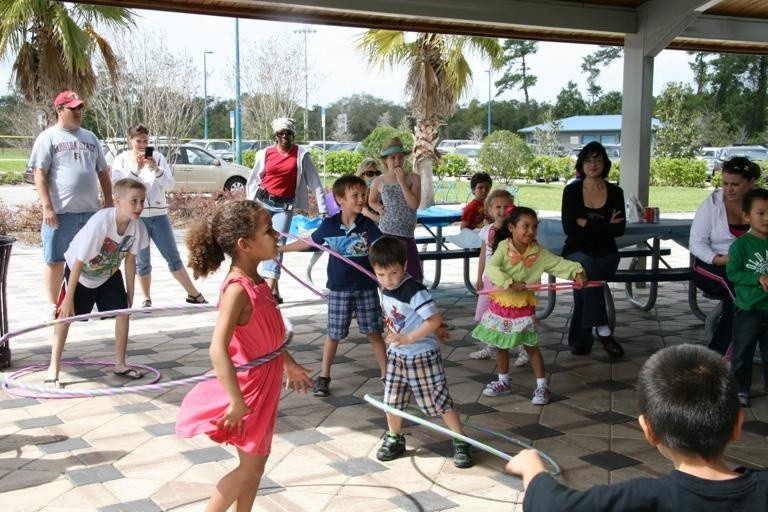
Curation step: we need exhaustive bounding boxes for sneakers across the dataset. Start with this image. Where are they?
[452,438,474,467]
[311,376,331,397]
[273,293,284,303]
[469,347,550,407]
[596,329,625,357]
[571,342,591,356]
[377,430,406,461]
[438,321,455,330]
[738,392,750,405]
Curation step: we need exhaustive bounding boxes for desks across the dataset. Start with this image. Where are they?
[536,216,721,322]
[417,206,464,290]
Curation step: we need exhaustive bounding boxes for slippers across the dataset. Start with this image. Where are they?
[44,377,62,390]
[113,364,143,380]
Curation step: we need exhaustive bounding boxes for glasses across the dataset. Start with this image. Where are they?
[64,106,82,111]
[365,170,381,177]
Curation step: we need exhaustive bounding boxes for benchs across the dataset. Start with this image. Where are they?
[278,244,330,287]
[615,246,672,258]
[702,292,767,367]
[415,236,446,272]
[608,268,695,282]
[417,248,481,297]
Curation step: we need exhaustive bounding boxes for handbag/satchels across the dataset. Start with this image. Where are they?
[627,193,643,223]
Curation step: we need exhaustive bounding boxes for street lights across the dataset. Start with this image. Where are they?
[200,50,215,140]
[484,70,491,137]
[292,27,318,141]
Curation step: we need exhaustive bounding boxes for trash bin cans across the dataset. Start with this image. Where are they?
[0,235,16,368]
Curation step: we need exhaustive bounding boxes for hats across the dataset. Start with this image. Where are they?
[382,146,411,158]
[55,91,84,108]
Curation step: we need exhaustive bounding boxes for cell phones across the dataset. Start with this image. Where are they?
[144,146,154,159]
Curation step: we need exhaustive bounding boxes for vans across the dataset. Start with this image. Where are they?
[97,140,251,205]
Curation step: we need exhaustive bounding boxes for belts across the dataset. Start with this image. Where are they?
[265,194,284,203]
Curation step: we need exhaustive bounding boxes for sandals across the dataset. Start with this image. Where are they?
[142,300,153,314]
[186,293,207,303]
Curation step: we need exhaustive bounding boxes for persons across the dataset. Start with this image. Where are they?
[354,157,384,227]
[368,138,422,284]
[727,189,768,406]
[367,236,472,469]
[459,171,493,230]
[277,172,391,396]
[688,157,768,357]
[27,90,113,318]
[483,207,588,405]
[247,117,329,304]
[562,139,626,358]
[42,178,150,384]
[173,200,314,512]
[469,189,530,367]
[109,122,208,316]
[501,344,768,510]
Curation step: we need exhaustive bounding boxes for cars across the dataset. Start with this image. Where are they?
[301,139,365,169]
[220,139,274,162]
[696,145,768,182]
[176,139,231,162]
[436,139,620,182]
